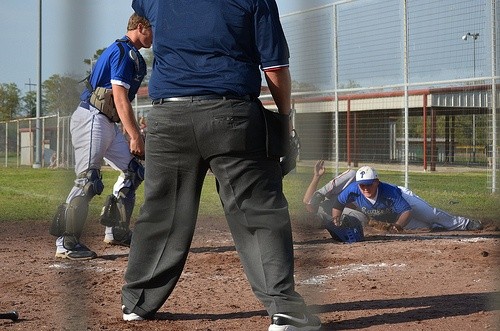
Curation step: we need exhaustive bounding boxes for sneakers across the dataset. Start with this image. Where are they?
[267,311,321,331]
[103,232,132,248]
[121,304,145,321]
[55,242,97,261]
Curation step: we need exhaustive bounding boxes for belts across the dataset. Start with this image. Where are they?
[153,94,249,104]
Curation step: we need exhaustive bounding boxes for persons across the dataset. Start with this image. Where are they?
[305,161,496,242]
[51,12,153,261]
[122,0,323,331]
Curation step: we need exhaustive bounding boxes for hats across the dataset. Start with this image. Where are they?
[355,166,377,186]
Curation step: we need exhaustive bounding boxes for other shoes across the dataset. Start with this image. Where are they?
[465,219,484,230]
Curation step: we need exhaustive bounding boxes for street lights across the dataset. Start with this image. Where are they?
[461,32,479,168]
